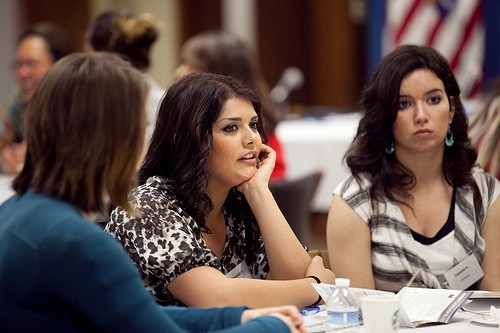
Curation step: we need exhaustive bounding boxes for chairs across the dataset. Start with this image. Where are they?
[268,171,322,249]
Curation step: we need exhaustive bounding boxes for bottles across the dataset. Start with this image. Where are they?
[326,278,360,333]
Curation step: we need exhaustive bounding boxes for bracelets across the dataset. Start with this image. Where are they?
[305,275,323,308]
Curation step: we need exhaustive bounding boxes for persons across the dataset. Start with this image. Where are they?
[104,71,338,311]
[0,19,79,179]
[325,45,500,295]
[0,52,304,333]
[83,10,165,183]
[174,30,286,182]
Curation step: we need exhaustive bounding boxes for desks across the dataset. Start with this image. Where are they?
[303,288,500,333]
[276,114,364,214]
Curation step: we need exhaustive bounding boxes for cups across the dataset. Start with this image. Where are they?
[359,296,398,333]
